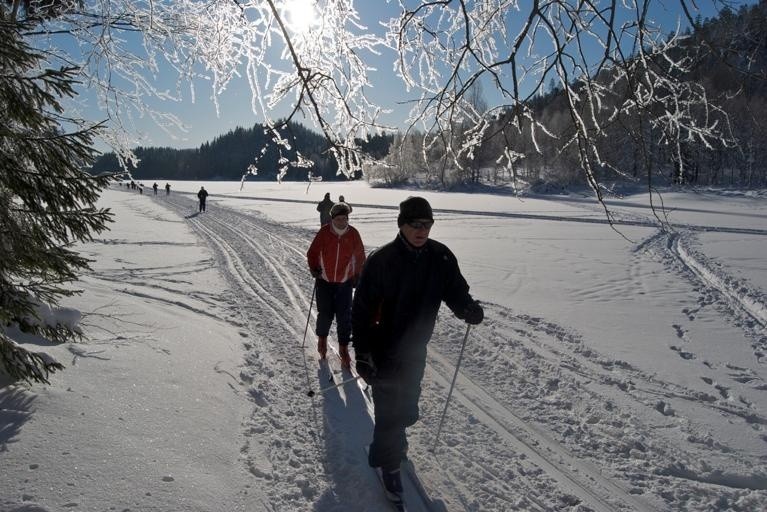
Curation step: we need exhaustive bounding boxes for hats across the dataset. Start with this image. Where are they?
[331,202,351,219]
[398,197,433,228]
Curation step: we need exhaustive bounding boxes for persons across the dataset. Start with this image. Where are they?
[164,182,171,196]
[315,191,334,228]
[196,187,209,212]
[306,202,367,371]
[152,182,158,196]
[118,181,145,196]
[348,195,485,503]
[332,195,351,218]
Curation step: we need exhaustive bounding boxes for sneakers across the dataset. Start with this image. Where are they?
[339,344,351,362]
[383,469,403,493]
[318,336,327,353]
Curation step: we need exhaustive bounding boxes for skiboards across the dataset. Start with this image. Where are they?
[365,444,433,512]
[308,323,371,393]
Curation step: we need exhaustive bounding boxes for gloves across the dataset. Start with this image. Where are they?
[352,273,360,288]
[356,359,376,385]
[310,265,323,278]
[465,301,484,324]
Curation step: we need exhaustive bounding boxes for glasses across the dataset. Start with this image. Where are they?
[408,220,433,229]
